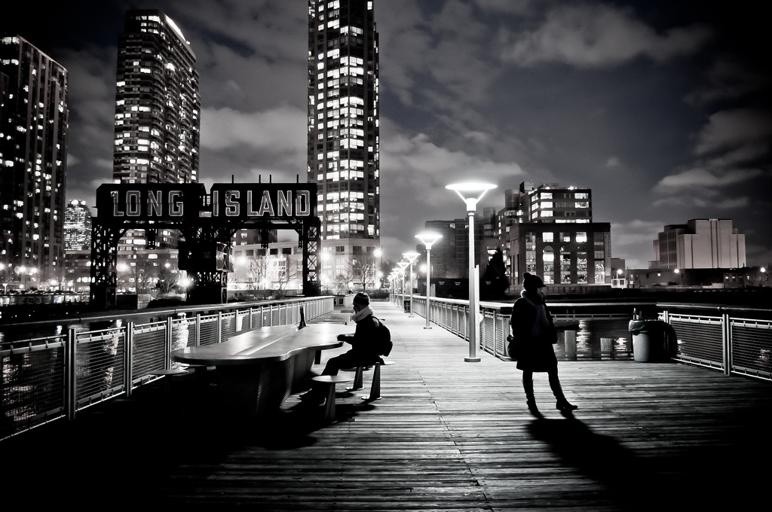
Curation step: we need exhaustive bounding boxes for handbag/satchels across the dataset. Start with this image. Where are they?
[506,335,521,360]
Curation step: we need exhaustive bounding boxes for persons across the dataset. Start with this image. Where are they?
[300,291,380,402]
[510,271,578,419]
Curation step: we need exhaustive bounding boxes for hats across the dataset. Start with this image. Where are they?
[353,292,370,305]
[523,272,547,290]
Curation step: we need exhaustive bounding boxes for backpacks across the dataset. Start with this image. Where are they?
[368,314,393,358]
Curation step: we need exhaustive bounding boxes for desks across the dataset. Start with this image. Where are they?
[160,321,348,443]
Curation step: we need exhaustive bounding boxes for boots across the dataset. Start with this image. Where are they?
[522,379,541,416]
[548,378,578,410]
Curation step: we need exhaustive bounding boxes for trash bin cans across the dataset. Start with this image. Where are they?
[404,300,410,312]
[631,319,664,362]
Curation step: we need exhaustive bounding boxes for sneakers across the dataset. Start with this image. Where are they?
[299,388,325,404]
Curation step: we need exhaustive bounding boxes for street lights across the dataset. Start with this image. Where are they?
[413,231,444,329]
[387,251,421,318]
[445,180,498,363]
[616,269,622,279]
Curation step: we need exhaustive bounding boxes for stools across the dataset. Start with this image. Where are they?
[311,358,384,422]
